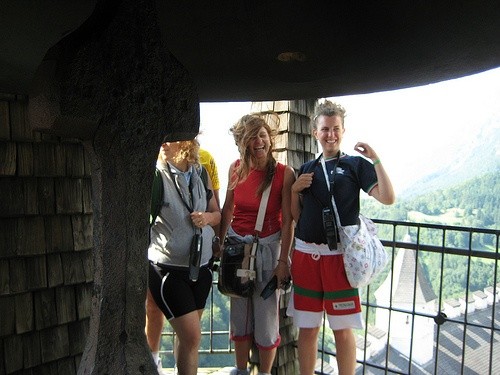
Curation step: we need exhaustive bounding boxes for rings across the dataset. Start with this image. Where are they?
[199,221,202,225]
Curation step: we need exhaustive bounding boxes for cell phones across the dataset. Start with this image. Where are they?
[323,209,336,231]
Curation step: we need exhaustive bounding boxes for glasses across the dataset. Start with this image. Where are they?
[279,275,291,290]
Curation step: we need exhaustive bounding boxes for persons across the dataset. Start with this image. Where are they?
[215,115,297,375]
[291,100,395,375]
[146,138,222,375]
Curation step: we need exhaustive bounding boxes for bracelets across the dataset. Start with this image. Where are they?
[212,235,220,243]
[277,258,288,264]
[372,159,381,166]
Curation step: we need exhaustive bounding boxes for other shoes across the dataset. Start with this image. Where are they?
[231,370,249,375]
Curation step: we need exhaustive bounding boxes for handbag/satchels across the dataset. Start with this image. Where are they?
[338,213,388,288]
[218,236,259,299]
